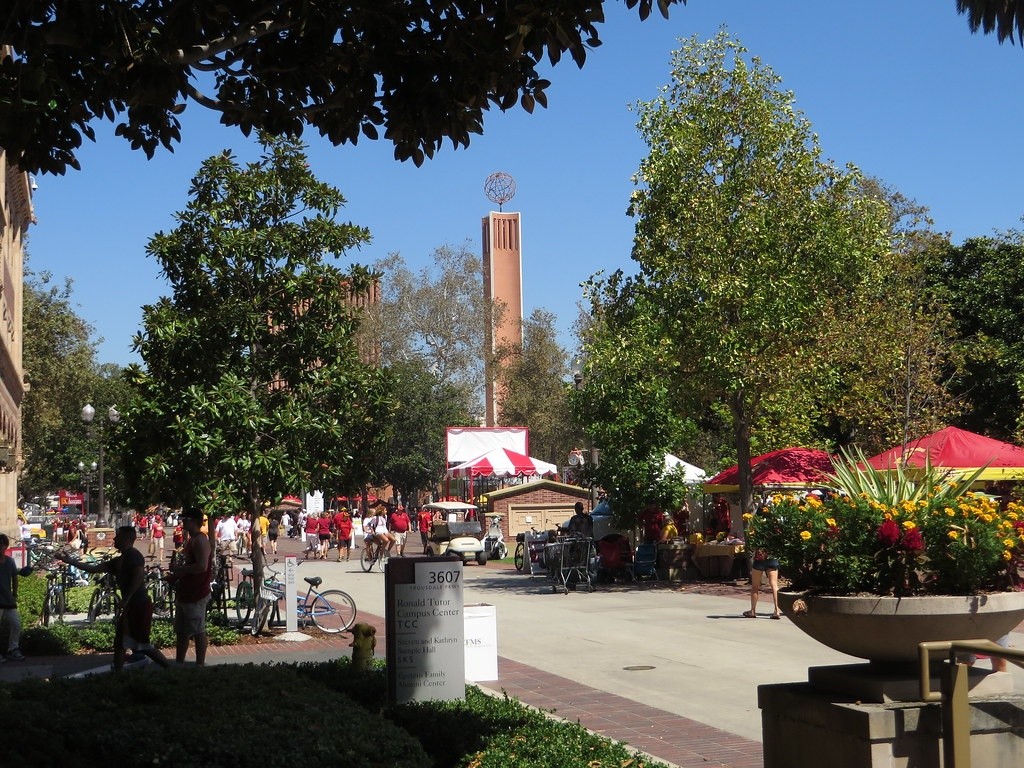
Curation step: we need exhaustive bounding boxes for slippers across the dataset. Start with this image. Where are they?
[743,611,756,618]
[770,614,780,619]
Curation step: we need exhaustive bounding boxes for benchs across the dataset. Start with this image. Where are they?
[432,525,466,542]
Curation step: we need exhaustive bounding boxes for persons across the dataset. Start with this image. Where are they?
[55,526,171,673]
[199,510,281,562]
[568,501,594,538]
[0,533,25,663]
[410,506,454,547]
[50,516,87,552]
[280,507,355,562]
[362,505,412,566]
[130,512,186,561]
[640,491,731,545]
[743,505,783,620]
[158,507,213,666]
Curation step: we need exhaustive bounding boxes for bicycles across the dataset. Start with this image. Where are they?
[514,526,545,570]
[360,531,392,573]
[15,537,357,634]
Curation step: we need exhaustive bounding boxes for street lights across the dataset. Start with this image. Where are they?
[80,403,120,529]
[77,460,98,529]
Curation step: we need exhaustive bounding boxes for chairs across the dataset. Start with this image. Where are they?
[597,540,659,584]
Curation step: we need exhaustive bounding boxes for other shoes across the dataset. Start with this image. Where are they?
[0,655,6,663]
[127,651,144,664]
[236,546,351,562]
[7,648,25,662]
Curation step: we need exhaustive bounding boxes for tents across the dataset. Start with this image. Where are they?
[445,448,558,518]
[702,446,845,543]
[847,425,1024,484]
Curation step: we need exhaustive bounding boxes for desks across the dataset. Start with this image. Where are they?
[692,542,747,579]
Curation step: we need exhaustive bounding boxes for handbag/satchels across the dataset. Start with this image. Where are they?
[180,571,202,601]
[127,605,152,643]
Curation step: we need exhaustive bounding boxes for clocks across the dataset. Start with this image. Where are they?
[568,454,579,465]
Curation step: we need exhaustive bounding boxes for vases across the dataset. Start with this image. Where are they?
[777,585,1024,663]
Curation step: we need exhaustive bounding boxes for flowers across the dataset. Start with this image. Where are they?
[743,436,1024,622]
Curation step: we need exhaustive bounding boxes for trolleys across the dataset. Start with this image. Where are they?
[543,535,594,595]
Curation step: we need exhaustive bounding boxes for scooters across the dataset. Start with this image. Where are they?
[480,514,508,560]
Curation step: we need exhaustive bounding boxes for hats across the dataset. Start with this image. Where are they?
[398,505,404,511]
[178,508,203,523]
